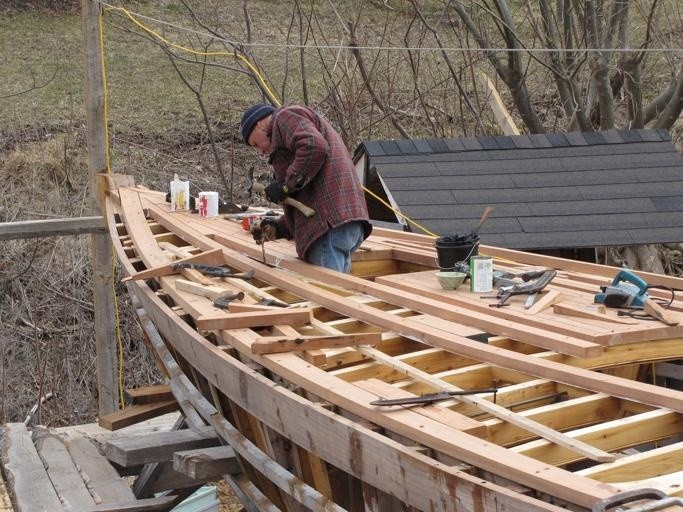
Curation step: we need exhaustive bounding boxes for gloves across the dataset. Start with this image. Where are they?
[250,216,292,244]
[265,180,292,205]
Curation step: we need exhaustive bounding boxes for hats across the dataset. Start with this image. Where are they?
[241,103,275,146]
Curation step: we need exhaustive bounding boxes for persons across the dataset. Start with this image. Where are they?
[242,103,371,274]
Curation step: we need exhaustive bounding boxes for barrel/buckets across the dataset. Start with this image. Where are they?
[432,234,481,283]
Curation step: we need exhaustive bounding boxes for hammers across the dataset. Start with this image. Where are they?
[242,166,316,217]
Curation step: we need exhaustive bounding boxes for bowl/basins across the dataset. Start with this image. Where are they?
[434,271,467,290]
